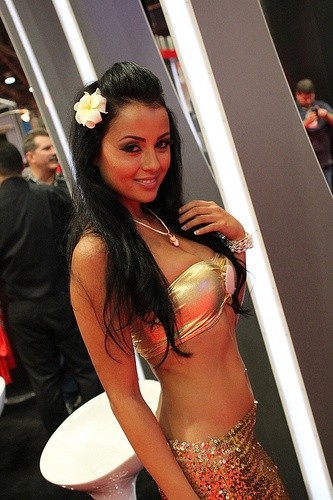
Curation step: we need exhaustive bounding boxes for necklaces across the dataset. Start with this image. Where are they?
[127,204,180,247]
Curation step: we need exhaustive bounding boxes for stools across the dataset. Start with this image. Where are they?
[40,379,161,500]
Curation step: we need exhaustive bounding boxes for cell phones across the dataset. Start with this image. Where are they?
[311,107,319,119]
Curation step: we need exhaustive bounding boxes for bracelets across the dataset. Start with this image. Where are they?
[217,229,254,254]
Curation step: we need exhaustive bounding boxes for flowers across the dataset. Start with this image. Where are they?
[73,88,109,129]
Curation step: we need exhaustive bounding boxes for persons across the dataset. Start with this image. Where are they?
[0,127,105,437]
[294,78,333,195]
[70,60,294,500]
